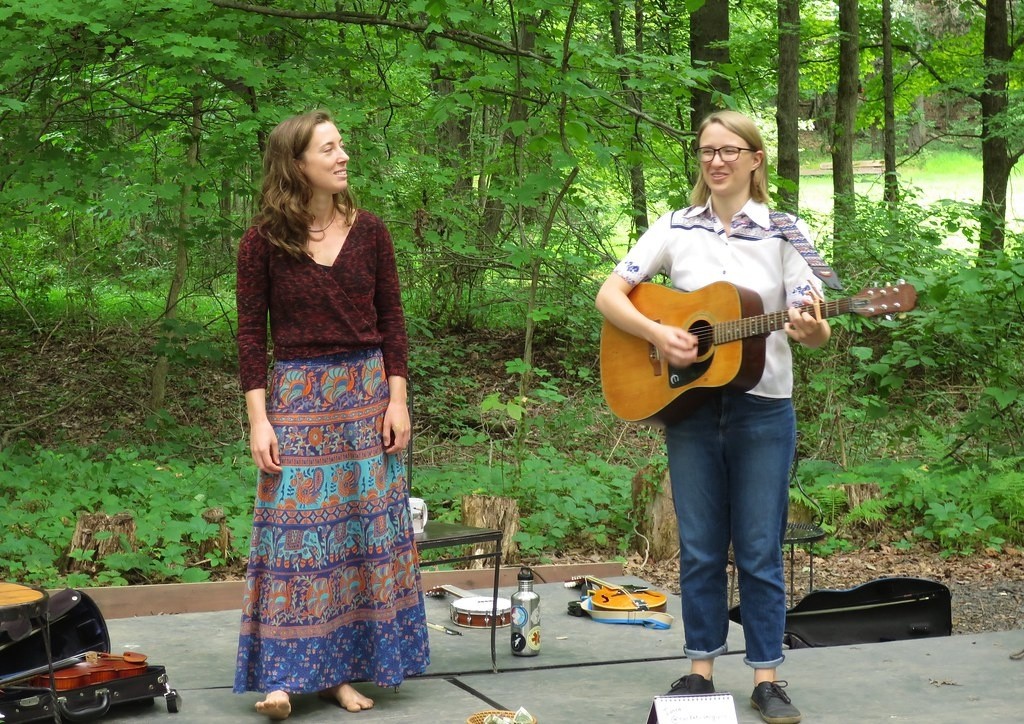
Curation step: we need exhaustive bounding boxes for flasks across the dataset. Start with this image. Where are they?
[510,566,542,657]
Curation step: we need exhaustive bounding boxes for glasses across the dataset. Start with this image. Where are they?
[695,146,757,163]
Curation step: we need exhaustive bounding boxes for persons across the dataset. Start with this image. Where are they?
[595,110,830,724]
[232,113,428,724]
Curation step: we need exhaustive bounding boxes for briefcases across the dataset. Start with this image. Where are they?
[0,588,183,724]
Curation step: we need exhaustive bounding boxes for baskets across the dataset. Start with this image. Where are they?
[467,710,538,724]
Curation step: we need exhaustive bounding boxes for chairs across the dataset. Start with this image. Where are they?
[392,374,504,694]
[726,446,828,610]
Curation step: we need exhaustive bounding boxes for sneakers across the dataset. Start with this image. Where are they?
[666,674,715,695]
[750,679,801,724]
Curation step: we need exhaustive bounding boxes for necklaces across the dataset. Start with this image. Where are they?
[310,210,336,232]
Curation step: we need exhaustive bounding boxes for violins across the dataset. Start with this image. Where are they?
[0,650,148,691]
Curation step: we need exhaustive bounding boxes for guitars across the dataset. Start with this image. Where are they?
[564,574,668,614]
[426,584,510,629]
[599,279,918,423]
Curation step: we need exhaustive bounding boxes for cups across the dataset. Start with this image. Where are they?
[408,497,428,534]
